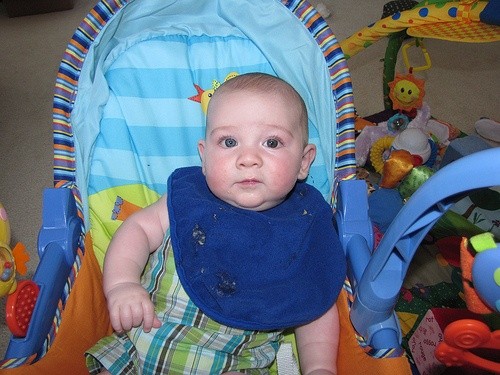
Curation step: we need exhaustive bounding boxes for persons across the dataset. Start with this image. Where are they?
[83,71,349,375]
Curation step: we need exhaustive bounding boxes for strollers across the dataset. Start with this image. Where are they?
[3,1,499,374]
